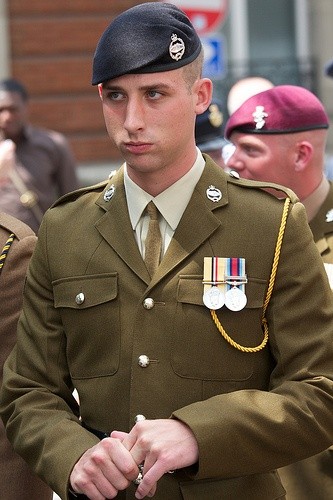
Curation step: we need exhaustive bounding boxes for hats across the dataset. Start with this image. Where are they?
[91,3,203,86]
[224,84,329,140]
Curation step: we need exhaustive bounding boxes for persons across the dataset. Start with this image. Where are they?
[0,211,54,500]
[0,80,80,238]
[0,1,333,500]
[196,63,333,287]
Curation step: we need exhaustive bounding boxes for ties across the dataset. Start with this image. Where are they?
[145,201,162,281]
